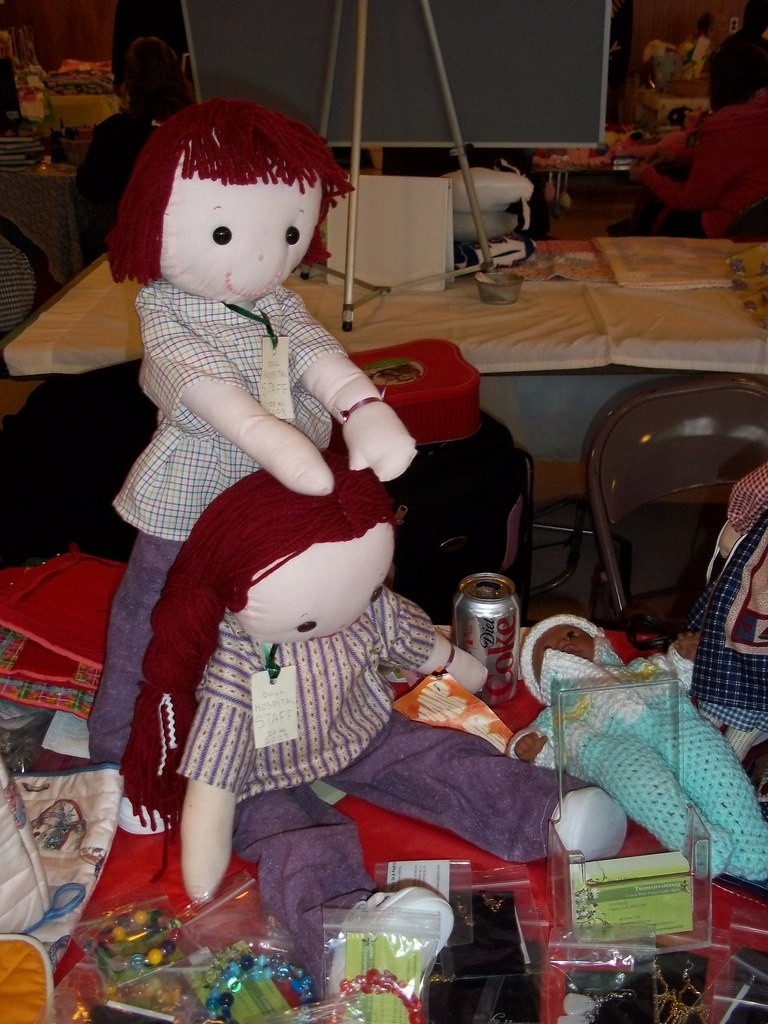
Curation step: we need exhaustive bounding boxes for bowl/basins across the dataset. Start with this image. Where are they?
[473,273,523,305]
[60,127,94,164]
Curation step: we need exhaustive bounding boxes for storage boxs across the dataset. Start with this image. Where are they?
[41,93,120,154]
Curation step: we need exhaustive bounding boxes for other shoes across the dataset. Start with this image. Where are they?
[605,218,652,236]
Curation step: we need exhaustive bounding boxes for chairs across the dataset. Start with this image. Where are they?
[372,372,768,639]
[0,214,67,381]
[719,193,768,236]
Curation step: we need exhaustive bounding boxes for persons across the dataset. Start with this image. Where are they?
[112,0,188,94]
[721,0,768,51]
[507,614,768,881]
[688,450,768,761]
[86,98,417,837]
[606,27,768,242]
[77,35,198,265]
[122,449,627,1001]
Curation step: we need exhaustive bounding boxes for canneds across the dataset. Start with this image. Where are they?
[451,572,522,706]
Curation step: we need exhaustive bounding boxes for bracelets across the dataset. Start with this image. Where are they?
[206,952,426,1024]
[96,910,181,972]
[441,640,455,673]
[340,397,381,422]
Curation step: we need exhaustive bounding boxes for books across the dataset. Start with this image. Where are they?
[0,133,47,172]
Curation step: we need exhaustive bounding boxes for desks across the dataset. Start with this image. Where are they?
[0,155,87,292]
[634,88,713,124]
[528,154,678,238]
[0,234,768,383]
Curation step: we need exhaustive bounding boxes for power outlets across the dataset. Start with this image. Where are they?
[728,17,738,35]
